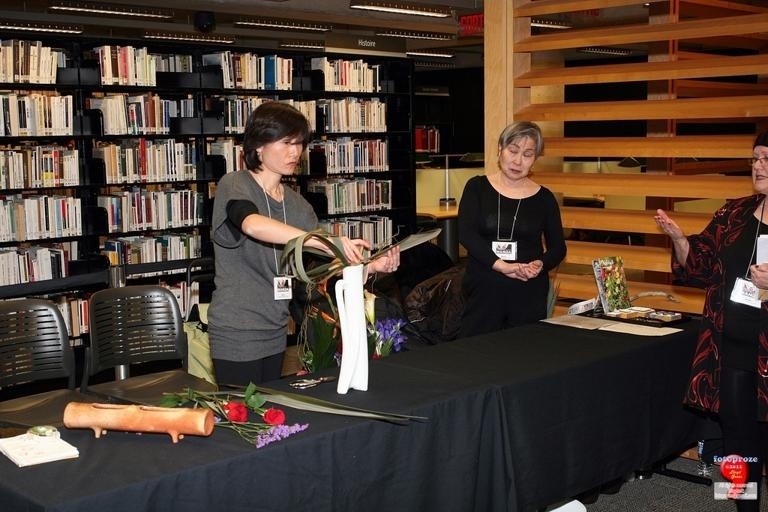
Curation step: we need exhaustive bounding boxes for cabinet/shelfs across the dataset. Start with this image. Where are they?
[0,26,423,402]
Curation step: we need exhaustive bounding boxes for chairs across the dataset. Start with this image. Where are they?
[76,282,218,409]
[182,254,219,324]
[366,240,455,298]
[0,296,120,434]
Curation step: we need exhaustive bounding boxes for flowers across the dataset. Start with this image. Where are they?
[153,382,312,451]
[330,314,412,367]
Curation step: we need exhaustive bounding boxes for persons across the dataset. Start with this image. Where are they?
[654,131,768,512]
[455,120,566,339]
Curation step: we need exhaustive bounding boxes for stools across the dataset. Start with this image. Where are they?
[280,334,312,378]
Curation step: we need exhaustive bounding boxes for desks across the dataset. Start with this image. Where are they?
[416,204,464,264]
[0,300,717,511]
